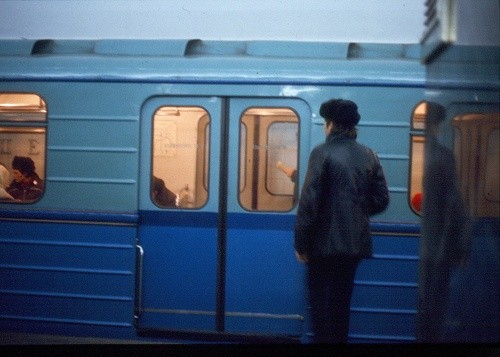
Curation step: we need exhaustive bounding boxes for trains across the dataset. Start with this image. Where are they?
[0,35,500,346]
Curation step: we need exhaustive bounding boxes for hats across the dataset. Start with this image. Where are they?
[319,98,361,125]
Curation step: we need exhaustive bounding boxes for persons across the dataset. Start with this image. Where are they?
[293,98,390,343]
[153,175,176,207]
[0,156,43,202]
[276,160,297,182]
